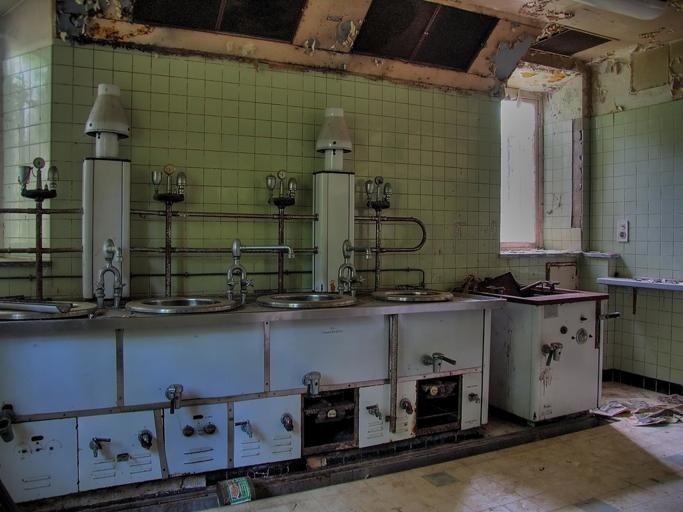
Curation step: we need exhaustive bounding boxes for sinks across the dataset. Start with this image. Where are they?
[368,287,455,301]
[0,294,99,320]
[126,293,240,313]
[255,291,358,308]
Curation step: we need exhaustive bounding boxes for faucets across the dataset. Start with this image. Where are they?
[0,403,17,442]
[103,237,124,265]
[366,403,384,420]
[421,352,456,373]
[281,416,295,432]
[165,383,185,416]
[544,260,579,281]
[346,243,372,260]
[138,431,154,451]
[469,392,481,404]
[399,398,416,414]
[542,343,558,368]
[232,237,295,261]
[88,435,111,458]
[234,418,254,439]
